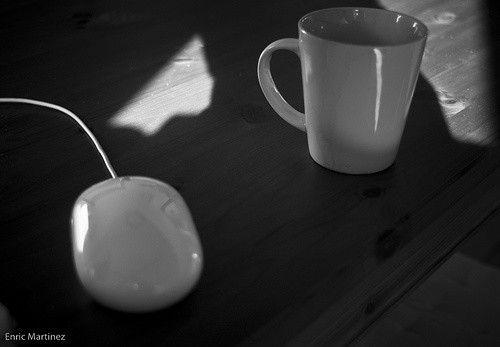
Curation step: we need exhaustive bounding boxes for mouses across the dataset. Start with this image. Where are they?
[70,172,204,312]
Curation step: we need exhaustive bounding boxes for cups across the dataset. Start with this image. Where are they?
[256,6,429,174]
